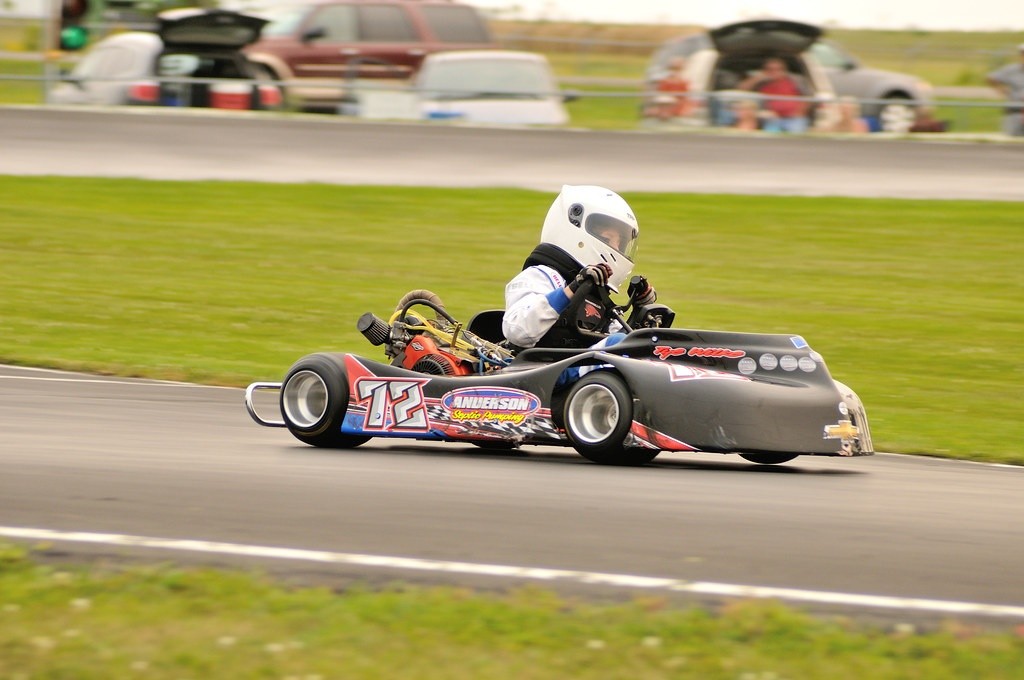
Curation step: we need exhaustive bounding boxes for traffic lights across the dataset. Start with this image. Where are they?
[53,0,93,54]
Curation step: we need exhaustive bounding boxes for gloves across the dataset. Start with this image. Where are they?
[635,276,658,308]
[576,262,613,287]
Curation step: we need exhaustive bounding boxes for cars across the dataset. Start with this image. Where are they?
[687,18,845,132]
[631,27,947,140]
[405,50,584,128]
[240,2,502,111]
[48,6,306,115]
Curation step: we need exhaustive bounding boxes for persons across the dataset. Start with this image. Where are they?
[986,43,1024,137]
[502,184,656,388]
[647,58,880,132]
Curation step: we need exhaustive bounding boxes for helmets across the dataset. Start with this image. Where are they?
[521,184,639,294]
